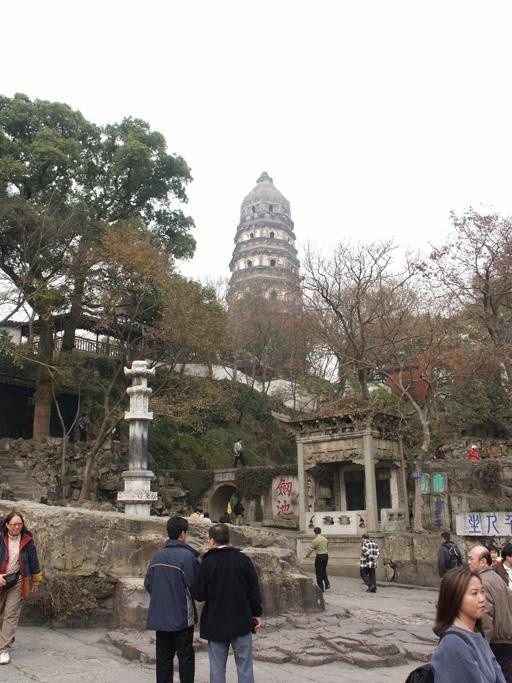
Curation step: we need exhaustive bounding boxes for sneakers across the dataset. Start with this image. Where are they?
[0,652,10,664]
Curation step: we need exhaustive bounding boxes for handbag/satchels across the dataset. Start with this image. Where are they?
[2,573,17,590]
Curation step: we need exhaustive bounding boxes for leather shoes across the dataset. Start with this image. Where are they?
[321,584,330,592]
[366,584,377,593]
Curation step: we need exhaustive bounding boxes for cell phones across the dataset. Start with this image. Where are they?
[252,616,257,633]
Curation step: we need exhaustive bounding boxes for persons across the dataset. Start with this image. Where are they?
[433,443,448,459]
[303,527,330,592]
[468,445,479,460]
[78,413,90,442]
[360,533,380,593]
[144,497,263,683]
[0,511,42,664]
[431,532,512,683]
[233,439,246,468]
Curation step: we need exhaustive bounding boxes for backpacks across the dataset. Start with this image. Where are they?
[405,630,469,683]
[442,544,462,568]
